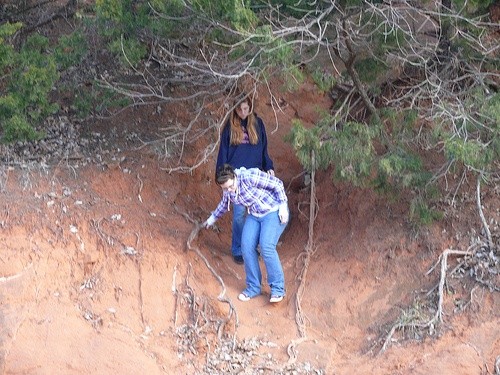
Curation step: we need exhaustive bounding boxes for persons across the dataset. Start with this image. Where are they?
[202,163,289,302]
[215,92,275,265]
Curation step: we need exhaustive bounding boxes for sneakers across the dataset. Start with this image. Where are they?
[238,292,251,303]
[234,256,244,264]
[270,294,283,303]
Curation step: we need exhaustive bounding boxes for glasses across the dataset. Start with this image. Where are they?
[223,179,234,190]
